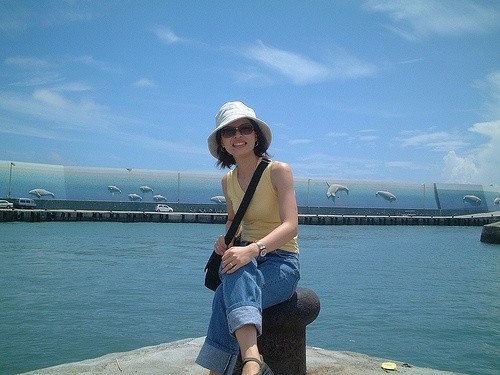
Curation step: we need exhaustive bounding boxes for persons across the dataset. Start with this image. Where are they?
[195,102,300,375]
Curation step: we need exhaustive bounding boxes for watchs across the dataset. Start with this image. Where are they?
[255,241,267,258]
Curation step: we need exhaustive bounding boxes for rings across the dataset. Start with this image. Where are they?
[229,262,234,267]
[216,246,219,249]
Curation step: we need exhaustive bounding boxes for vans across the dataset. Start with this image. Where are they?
[13,197,37,210]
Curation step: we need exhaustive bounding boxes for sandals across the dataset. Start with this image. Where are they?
[240,354,274,375]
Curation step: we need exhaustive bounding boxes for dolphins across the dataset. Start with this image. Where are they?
[326,182,349,199]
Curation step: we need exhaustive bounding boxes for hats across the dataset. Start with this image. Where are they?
[207,101,272,160]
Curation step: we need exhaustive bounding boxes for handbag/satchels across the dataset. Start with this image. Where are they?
[205,239,222,291]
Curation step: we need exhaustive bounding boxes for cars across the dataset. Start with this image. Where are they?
[155,203,173,213]
[0,200,14,209]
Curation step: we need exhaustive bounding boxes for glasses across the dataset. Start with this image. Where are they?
[219,123,256,138]
